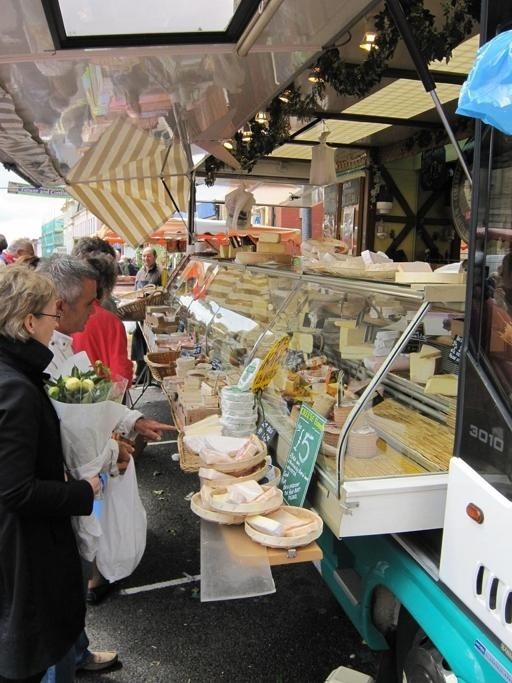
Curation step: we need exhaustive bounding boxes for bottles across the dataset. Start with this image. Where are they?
[321,400,380,457]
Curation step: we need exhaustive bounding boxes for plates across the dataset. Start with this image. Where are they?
[190,435,324,548]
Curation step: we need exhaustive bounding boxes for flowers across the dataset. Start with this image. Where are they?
[42,359,125,561]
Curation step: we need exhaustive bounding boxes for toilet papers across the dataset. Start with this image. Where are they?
[195,218,228,236]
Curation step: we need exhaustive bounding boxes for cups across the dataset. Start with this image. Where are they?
[220,244,253,258]
[409,352,436,385]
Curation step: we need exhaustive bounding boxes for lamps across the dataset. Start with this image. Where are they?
[358,14,379,51]
[307,46,340,84]
[220,79,295,150]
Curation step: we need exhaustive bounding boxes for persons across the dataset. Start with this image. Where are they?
[1,230,178,681]
[1,0,250,172]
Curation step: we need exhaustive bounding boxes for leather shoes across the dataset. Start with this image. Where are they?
[75,649,118,672]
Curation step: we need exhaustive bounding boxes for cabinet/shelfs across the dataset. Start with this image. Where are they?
[164,252,466,541]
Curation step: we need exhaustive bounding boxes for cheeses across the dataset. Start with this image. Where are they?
[288,317,459,396]
[220,232,286,259]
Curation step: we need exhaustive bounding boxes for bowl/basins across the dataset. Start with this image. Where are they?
[236,251,272,264]
[175,357,227,399]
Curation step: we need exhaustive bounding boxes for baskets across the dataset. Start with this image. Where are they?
[144,351,180,382]
[113,284,169,321]
[177,427,284,527]
[243,504,324,550]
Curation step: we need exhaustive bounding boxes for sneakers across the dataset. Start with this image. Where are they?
[86,582,115,602]
